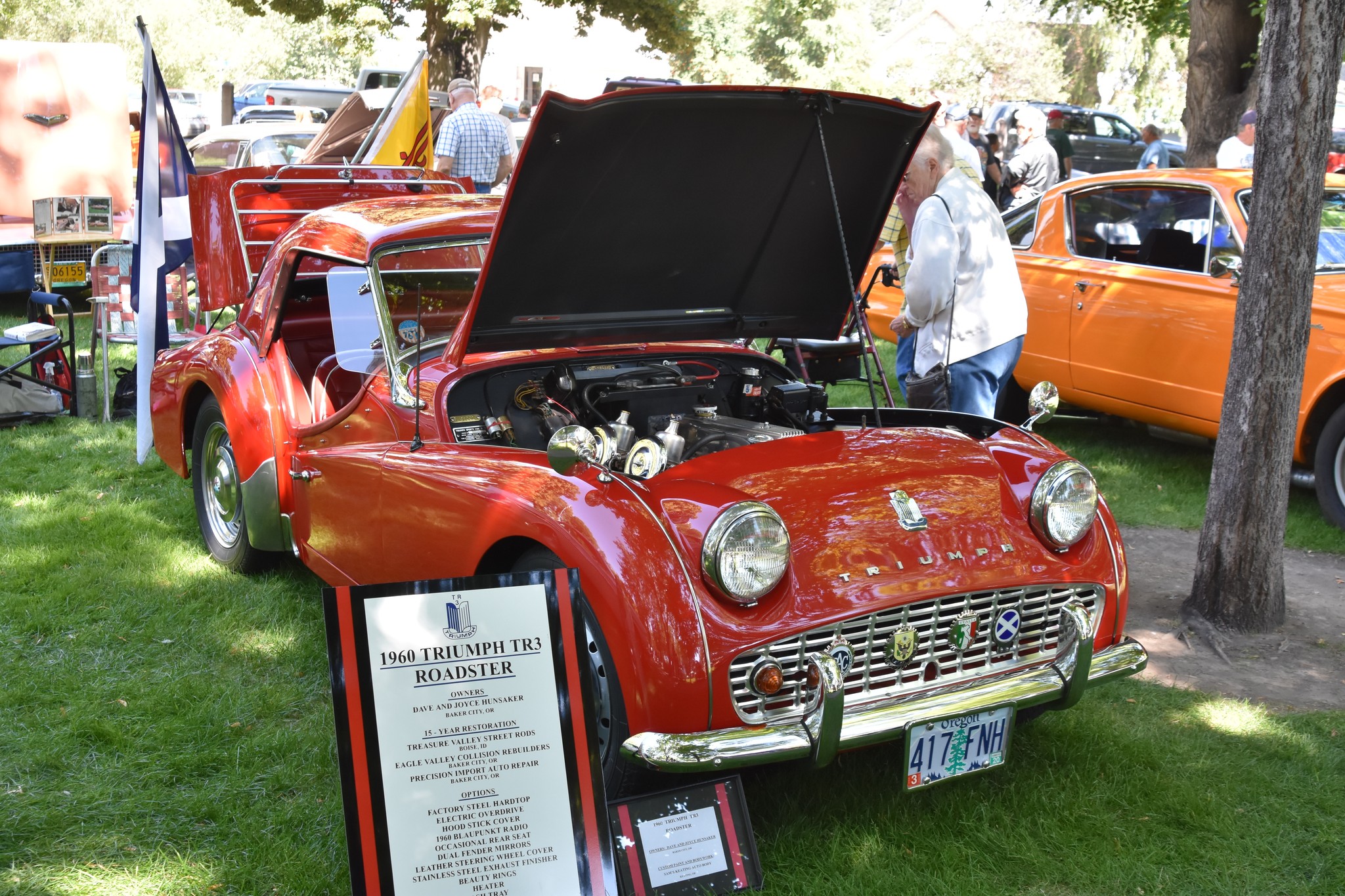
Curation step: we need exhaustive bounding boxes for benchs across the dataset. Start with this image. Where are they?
[280,290,475,413]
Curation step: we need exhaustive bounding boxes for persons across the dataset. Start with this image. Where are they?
[433,78,513,194]
[511,101,531,123]
[878,103,1257,420]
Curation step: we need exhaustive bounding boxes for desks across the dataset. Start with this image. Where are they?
[34,232,113,322]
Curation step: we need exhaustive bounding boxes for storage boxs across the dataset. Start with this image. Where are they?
[776,340,863,383]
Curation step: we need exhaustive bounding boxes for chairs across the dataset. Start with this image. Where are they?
[90,245,214,423]
[1140,228,1194,272]
[0,250,77,423]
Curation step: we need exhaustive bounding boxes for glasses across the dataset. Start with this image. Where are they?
[902,161,927,182]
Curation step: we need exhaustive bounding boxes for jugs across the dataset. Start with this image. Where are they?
[76,352,99,422]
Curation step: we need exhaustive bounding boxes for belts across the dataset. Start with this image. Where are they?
[474,182,491,185]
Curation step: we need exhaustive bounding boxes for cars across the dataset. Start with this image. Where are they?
[166,70,534,196]
[151,74,1149,807]
[854,168,1345,534]
[1326,127,1345,174]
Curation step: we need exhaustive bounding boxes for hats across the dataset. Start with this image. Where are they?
[447,78,474,106]
[969,107,982,117]
[519,101,531,112]
[946,104,969,121]
[1238,110,1256,125]
[1048,111,1069,119]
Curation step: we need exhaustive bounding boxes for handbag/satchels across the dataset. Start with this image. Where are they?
[905,362,951,412]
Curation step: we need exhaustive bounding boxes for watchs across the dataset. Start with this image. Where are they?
[902,314,918,330]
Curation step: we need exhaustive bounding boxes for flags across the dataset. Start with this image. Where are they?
[129,27,198,465]
[359,50,434,172]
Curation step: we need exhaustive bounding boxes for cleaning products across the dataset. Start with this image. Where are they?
[42,360,70,412]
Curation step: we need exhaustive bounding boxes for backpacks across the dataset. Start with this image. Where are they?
[112,363,137,422]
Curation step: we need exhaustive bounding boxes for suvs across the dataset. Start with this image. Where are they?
[981,99,1186,176]
